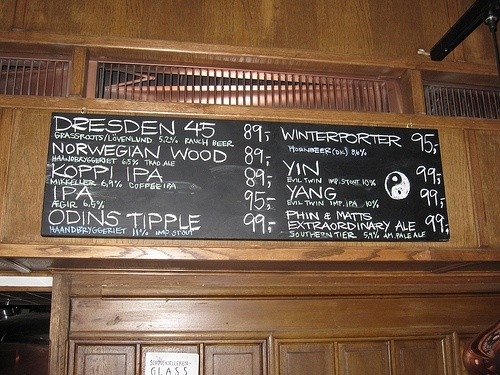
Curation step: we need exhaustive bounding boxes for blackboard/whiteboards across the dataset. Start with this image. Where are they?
[40,110,452,243]
